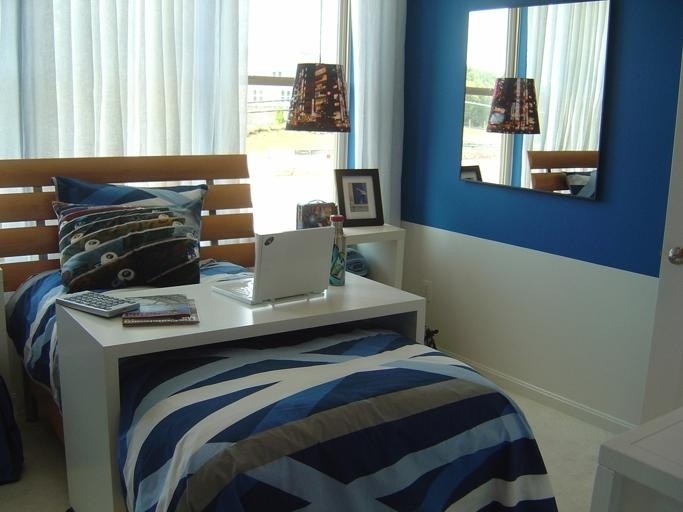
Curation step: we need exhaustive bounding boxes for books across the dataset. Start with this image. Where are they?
[121,297,199,326]
[121,292,190,319]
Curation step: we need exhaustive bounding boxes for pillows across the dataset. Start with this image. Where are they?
[561,169,598,200]
[46,174,210,296]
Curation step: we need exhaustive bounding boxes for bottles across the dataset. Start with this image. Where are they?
[327,214,348,287]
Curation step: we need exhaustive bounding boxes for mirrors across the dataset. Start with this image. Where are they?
[456,1,612,201]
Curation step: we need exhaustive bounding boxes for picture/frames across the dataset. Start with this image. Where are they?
[334,165,386,229]
[460,164,483,182]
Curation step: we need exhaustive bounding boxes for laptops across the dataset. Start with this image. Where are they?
[211,225,336,306]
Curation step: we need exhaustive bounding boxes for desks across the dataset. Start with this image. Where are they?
[53,272,429,510]
[342,223,407,289]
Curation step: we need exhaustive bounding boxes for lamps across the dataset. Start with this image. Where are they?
[485,8,541,136]
[284,0,352,136]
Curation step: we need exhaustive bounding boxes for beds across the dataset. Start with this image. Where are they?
[0,151,558,511]
